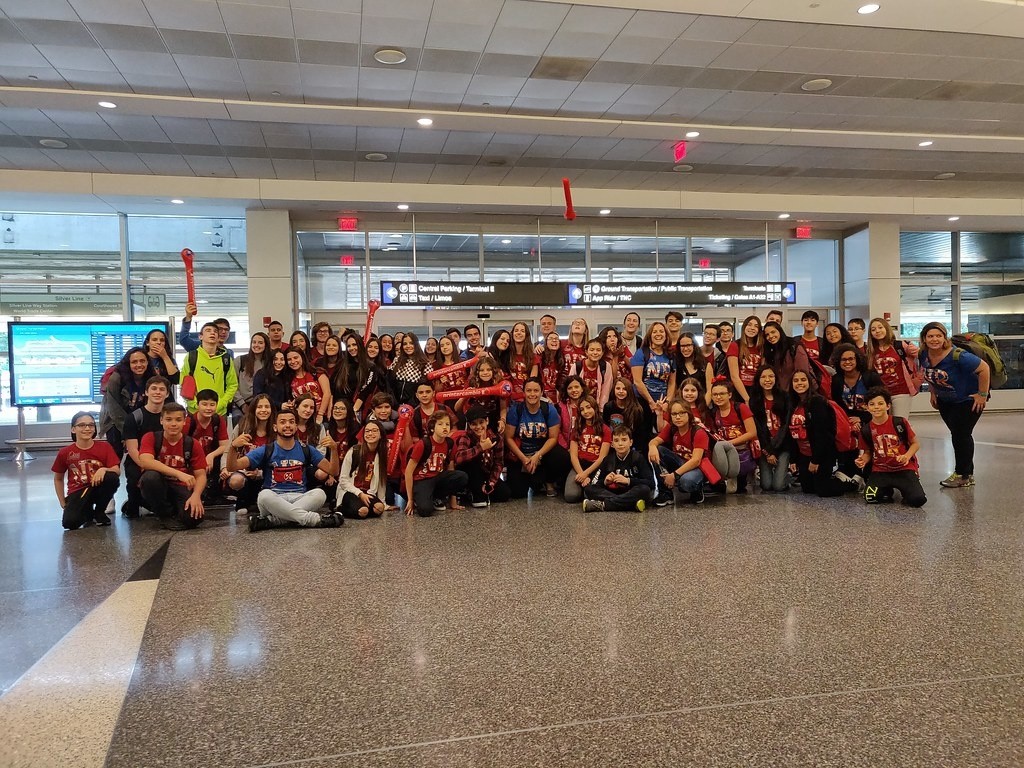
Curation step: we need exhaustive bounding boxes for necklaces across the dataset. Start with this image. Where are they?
[623,332,633,346]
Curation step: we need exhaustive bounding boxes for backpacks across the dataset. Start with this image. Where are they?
[918,331,1007,389]
[828,398,857,452]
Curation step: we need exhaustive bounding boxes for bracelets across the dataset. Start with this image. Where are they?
[231,442,236,449]
[978,391,988,396]
[319,410,325,413]
[628,477,629,485]
[317,413,323,416]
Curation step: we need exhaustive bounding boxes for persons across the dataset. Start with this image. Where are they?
[918,322,990,487]
[99,302,927,533]
[50,411,121,530]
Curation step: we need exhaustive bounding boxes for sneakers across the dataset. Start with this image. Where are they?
[433,500,447,510]
[248,514,274,532]
[83,508,94,528]
[633,499,646,513]
[316,510,343,528]
[105,498,115,513]
[968,474,975,484]
[652,491,675,506]
[204,493,236,510]
[850,474,865,493]
[835,471,852,485]
[940,472,970,488]
[92,510,111,526]
[690,483,704,504]
[582,499,605,513]
[472,488,487,507]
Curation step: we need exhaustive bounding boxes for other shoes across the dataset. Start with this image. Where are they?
[542,481,557,496]
[163,515,186,531]
[122,500,140,519]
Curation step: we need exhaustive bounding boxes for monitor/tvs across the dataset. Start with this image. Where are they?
[8,322,169,407]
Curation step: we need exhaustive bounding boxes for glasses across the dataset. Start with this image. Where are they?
[841,356,856,363]
[364,428,380,434]
[73,422,96,430]
[332,406,347,412]
[670,410,688,418]
[849,327,863,331]
[680,343,694,350]
[711,392,729,397]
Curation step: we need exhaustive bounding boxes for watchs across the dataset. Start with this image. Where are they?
[674,471,681,480]
